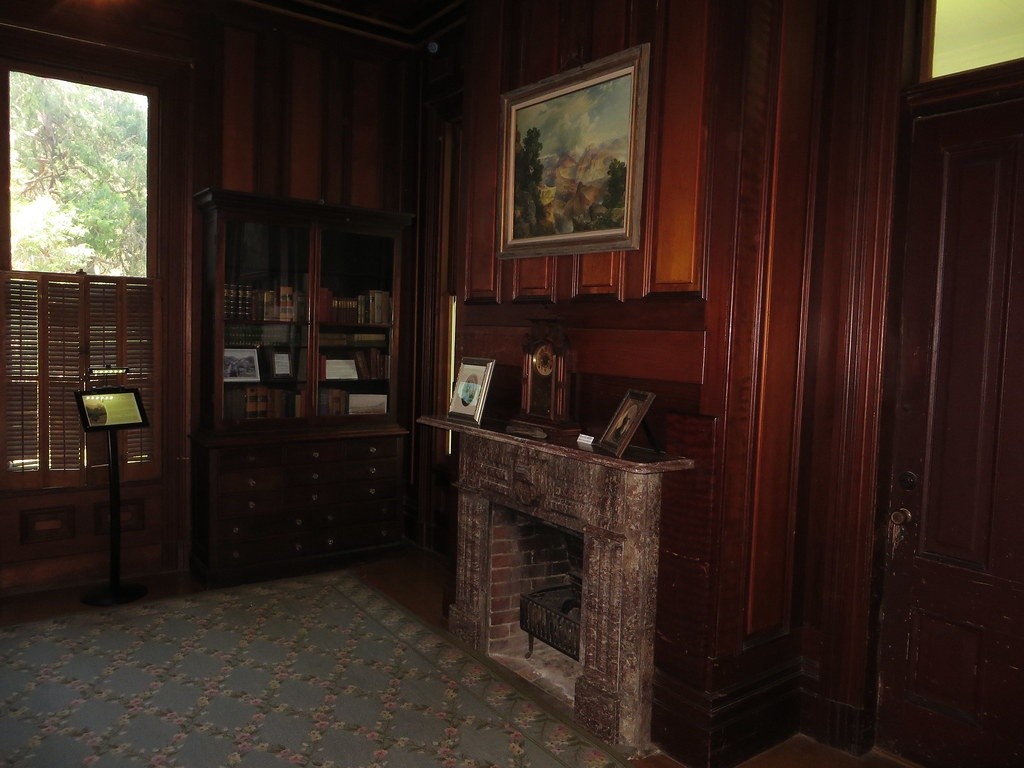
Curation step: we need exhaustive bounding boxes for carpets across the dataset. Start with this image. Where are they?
[0,570,636,768]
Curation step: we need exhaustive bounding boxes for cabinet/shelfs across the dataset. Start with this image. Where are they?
[194,188,411,586]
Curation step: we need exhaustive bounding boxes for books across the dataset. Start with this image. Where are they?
[318,385,348,416]
[319,286,389,323]
[319,348,390,379]
[224,323,300,347]
[319,332,388,348]
[223,282,306,322]
[224,386,300,420]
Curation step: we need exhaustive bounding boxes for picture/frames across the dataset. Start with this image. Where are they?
[494,41,652,260]
[223,345,263,385]
[598,388,657,458]
[447,357,496,426]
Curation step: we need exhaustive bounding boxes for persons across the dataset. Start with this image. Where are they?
[461,377,476,406]
[614,412,631,440]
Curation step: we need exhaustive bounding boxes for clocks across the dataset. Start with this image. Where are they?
[508,303,583,437]
